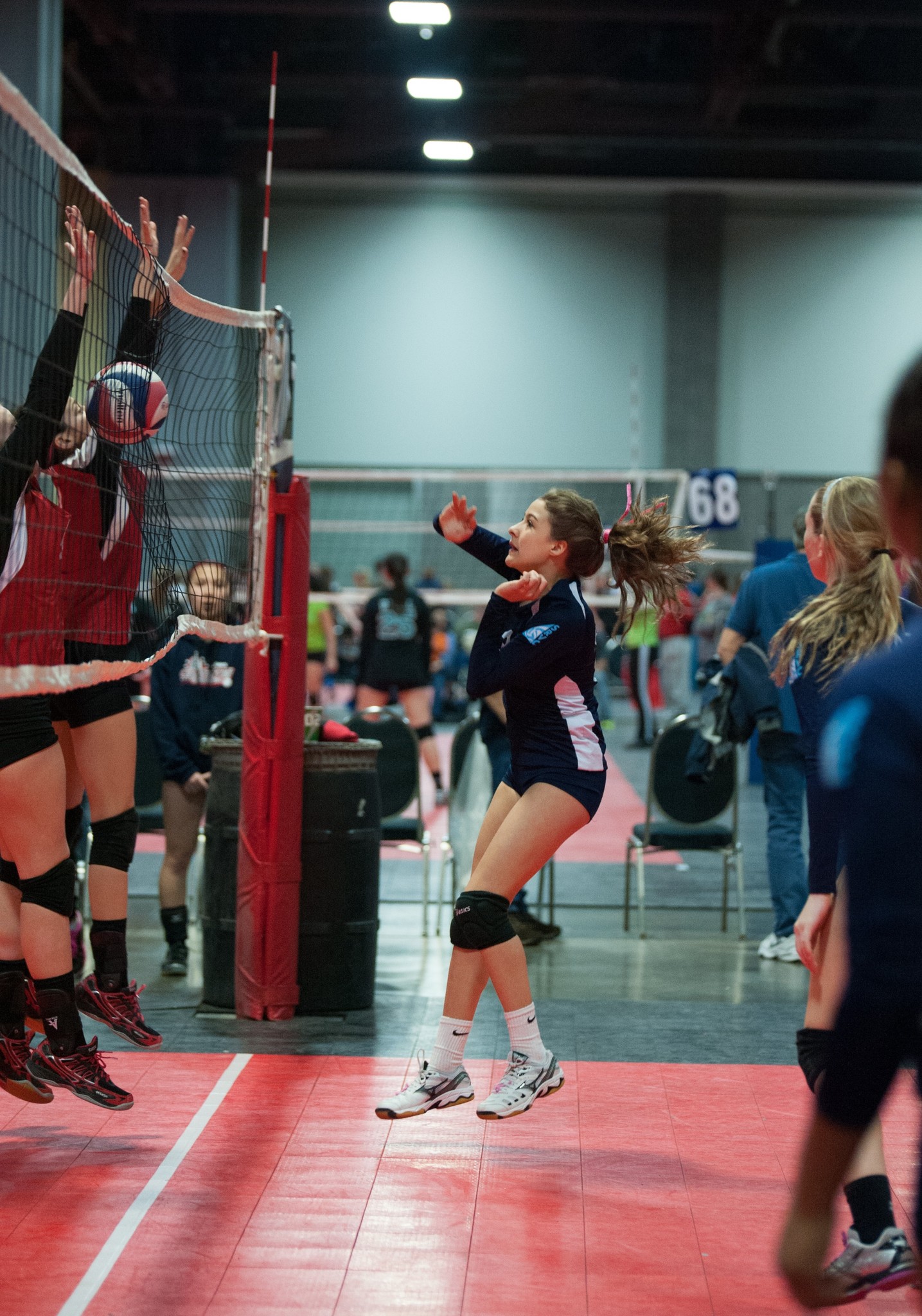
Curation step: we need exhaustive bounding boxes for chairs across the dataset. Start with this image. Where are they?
[435,712,556,938]
[623,711,748,943]
[341,705,431,940]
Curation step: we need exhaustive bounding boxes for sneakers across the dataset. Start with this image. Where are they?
[477,1049,564,1119]
[506,891,561,946]
[435,787,447,807]
[0,1030,54,1104]
[24,980,46,1034]
[69,909,86,977]
[75,974,163,1050]
[161,944,189,975]
[756,932,801,962]
[823,1224,919,1302]
[374,1049,474,1119]
[24,1037,134,1111]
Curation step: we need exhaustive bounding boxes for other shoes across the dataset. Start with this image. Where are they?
[625,737,658,749]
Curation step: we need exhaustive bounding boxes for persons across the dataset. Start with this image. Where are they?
[0,186,922,1316]
[374,483,714,1118]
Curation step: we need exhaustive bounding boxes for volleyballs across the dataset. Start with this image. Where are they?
[86,360,168,444]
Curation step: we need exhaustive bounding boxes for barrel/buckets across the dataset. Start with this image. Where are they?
[197,734,384,1017]
